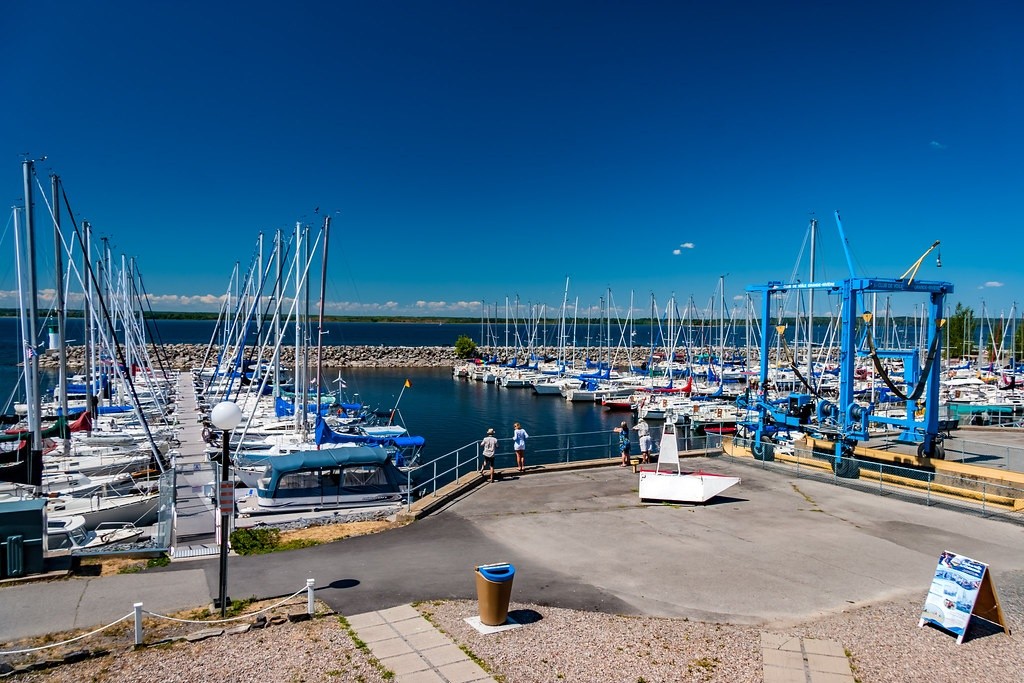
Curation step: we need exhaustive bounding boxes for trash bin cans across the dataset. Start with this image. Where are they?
[474,562,516,626]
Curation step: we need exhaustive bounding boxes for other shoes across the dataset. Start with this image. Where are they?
[516,467,522,470]
[521,467,526,471]
[491,480,494,483]
[642,460,646,464]
[480,470,483,473]
[620,464,626,467]
[647,461,650,464]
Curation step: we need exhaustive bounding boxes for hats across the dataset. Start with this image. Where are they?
[487,428,495,433]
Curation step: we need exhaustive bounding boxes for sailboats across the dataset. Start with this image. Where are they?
[199,211,422,527]
[450,278,1023,431]
[0,158,170,576]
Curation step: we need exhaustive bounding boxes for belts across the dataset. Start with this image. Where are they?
[639,434,649,438]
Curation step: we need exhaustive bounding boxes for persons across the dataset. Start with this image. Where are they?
[513,423,529,471]
[479,429,498,483]
[613,421,631,467]
[633,418,652,464]
